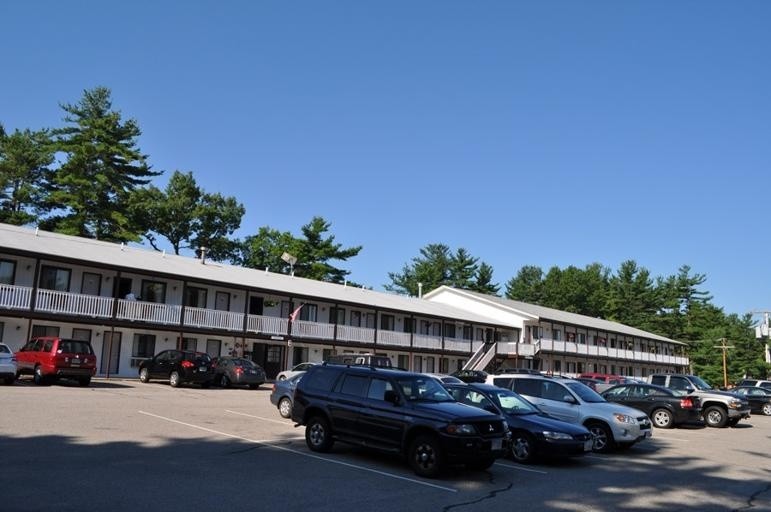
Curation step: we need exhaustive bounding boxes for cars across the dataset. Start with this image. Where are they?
[137,348,219,389]
[0,341,17,384]
[209,355,266,388]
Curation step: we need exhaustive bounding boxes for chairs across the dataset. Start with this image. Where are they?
[464,398,498,412]
[62,345,69,352]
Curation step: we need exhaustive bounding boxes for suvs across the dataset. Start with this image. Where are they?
[13,335,99,387]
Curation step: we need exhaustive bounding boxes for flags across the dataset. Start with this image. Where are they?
[290,302,305,322]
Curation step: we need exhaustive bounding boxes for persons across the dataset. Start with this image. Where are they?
[727,382,737,390]
